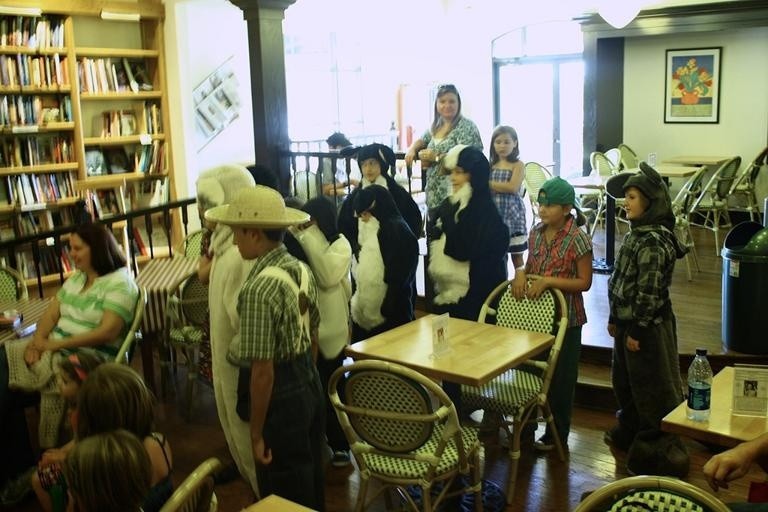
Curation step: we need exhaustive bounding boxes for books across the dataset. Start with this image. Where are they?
[2,15,84,282]
[92,100,163,138]
[76,55,153,94]
[85,140,167,175]
[91,178,171,258]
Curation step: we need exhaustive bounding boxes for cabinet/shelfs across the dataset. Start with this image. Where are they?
[1,0,86,288]
[60,0,184,264]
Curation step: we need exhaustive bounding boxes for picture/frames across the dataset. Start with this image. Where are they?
[662,46,722,124]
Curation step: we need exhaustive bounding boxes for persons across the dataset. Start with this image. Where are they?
[703,433,767,492]
[196,135,423,512]
[605,163,691,480]
[503,177,591,451]
[406,84,483,215]
[24,216,174,512]
[489,126,529,268]
[425,144,509,326]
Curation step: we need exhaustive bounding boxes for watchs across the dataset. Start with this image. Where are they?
[435,150,441,165]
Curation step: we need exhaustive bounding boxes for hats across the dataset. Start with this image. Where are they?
[537,176,574,205]
[205,186,311,227]
[326,133,353,148]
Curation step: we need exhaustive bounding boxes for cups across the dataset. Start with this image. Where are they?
[649,153,656,169]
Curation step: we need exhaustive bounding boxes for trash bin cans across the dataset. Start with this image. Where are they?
[720,221,768,355]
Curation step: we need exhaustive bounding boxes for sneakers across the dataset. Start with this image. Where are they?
[331,448,353,467]
[606,426,637,450]
[534,433,569,452]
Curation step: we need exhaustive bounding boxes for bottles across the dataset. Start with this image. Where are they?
[686,348,713,422]
[390,122,397,152]
